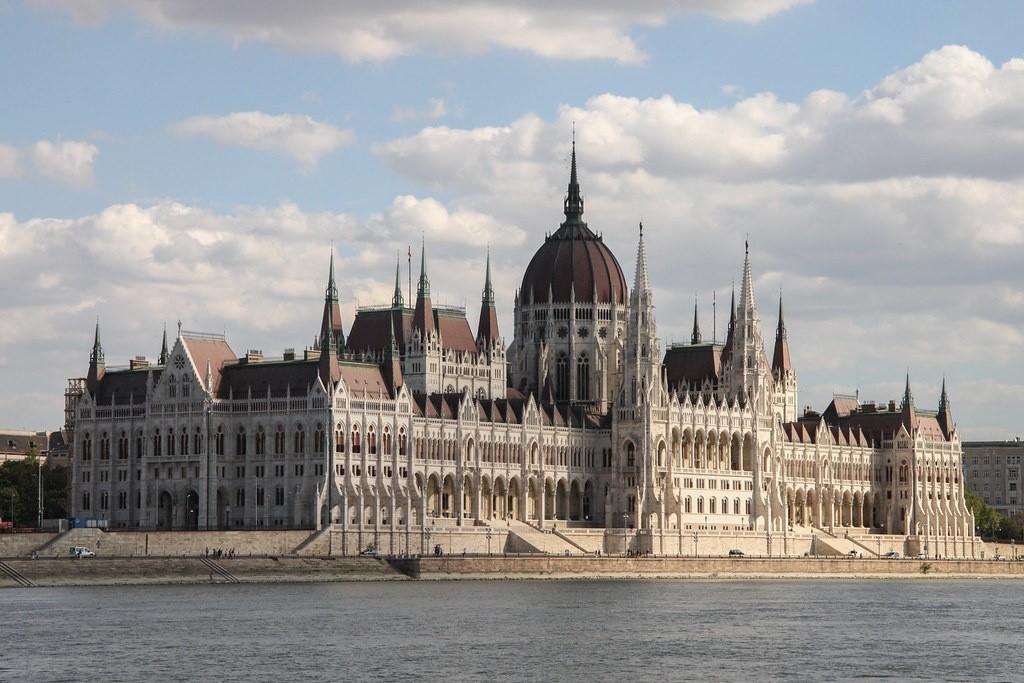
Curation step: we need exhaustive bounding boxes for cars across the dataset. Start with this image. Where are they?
[730,549,745,558]
[881,552,899,560]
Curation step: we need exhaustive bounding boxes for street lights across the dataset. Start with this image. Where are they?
[12,493,15,531]
[251,474,258,531]
[37,462,43,528]
[622,509,629,557]
[265,492,272,528]
[185,494,191,531]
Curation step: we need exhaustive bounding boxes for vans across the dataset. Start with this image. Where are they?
[69,546,95,558]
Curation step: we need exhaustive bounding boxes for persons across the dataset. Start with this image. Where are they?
[205,547,236,560]
[627,548,649,557]
[935,554,941,560]
[434,544,443,558]
[595,549,601,557]
[31,551,35,561]
[78,549,82,560]
[35,549,40,561]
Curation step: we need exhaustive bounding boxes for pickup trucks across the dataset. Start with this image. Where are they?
[0,516,17,533]
